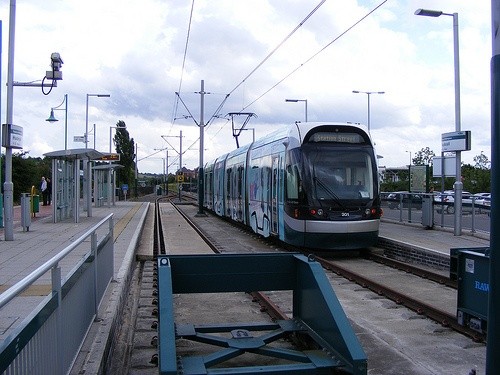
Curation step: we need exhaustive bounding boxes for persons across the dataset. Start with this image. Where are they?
[250,167,269,214]
[41,177,52,206]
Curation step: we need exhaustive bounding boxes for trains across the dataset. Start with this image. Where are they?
[182,124,383,253]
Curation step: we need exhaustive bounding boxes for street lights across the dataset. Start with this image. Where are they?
[416,8,465,235]
[83,95,111,215]
[286,99,308,123]
[354,89,384,133]
[45,93,68,152]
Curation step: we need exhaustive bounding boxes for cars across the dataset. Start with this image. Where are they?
[390,192,424,211]
[427,190,492,209]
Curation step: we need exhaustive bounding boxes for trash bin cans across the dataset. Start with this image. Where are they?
[28,194,40,218]
[21,193,31,232]
